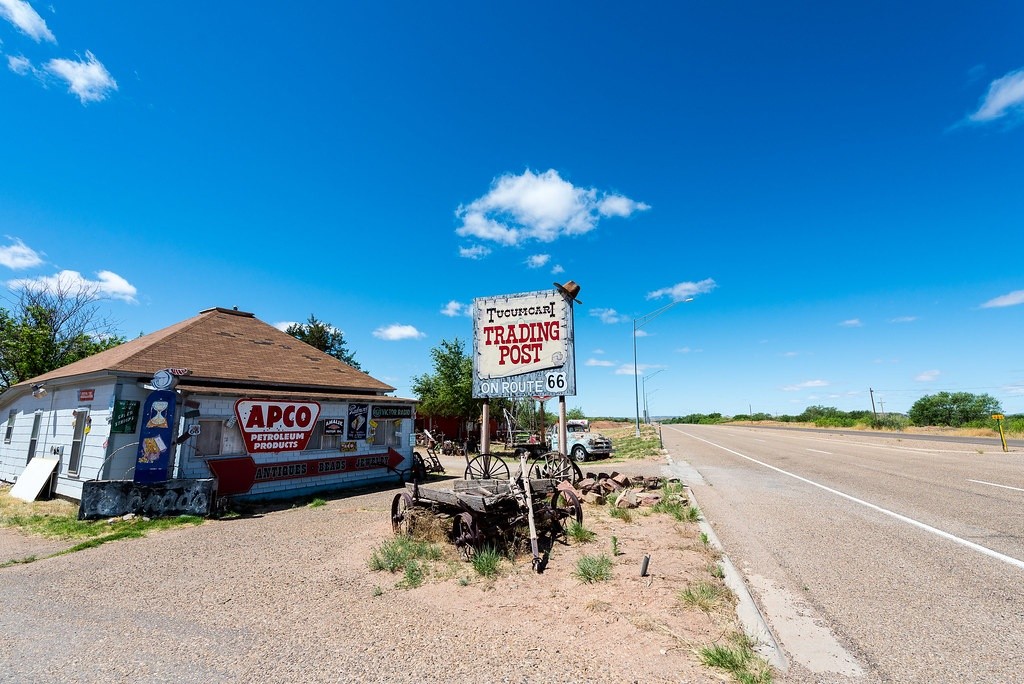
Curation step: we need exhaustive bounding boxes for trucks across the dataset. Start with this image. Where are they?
[514,422,612,462]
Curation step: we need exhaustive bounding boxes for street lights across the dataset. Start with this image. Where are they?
[643,369,668,424]
[633,298,694,428]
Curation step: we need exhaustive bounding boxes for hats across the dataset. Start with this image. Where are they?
[553,280,582,304]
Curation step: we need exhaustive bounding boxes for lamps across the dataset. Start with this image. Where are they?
[30,383,49,399]
